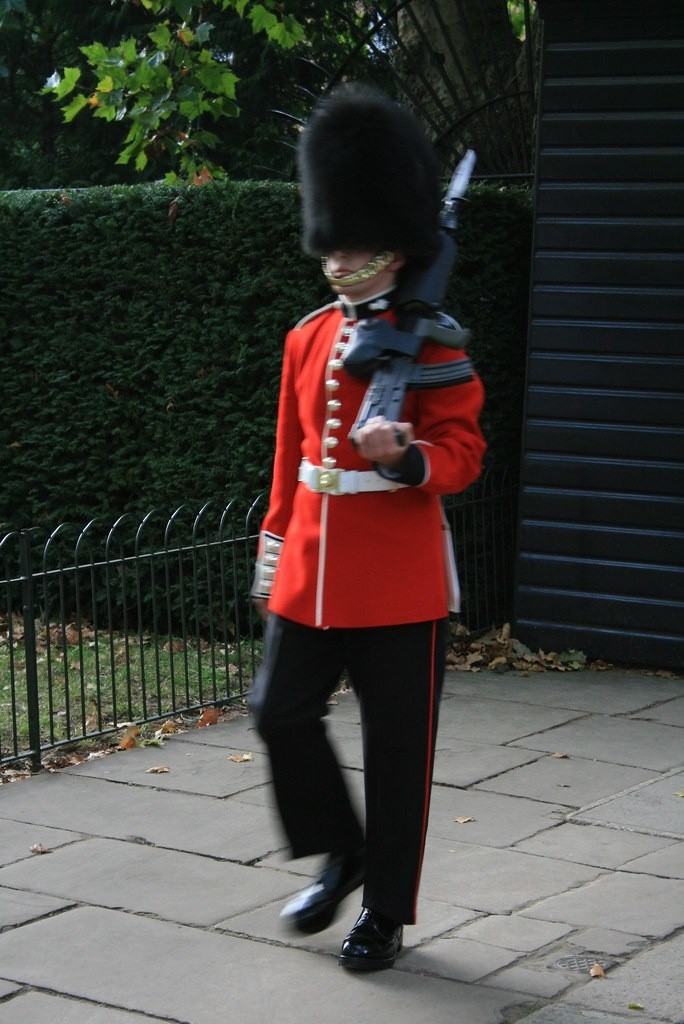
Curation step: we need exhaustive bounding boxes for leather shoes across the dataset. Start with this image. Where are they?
[278,854,367,935]
[338,906,409,969]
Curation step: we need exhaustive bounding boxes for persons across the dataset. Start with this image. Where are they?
[247,84,486,971]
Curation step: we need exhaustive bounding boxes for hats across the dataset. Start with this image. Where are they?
[301,86,438,269]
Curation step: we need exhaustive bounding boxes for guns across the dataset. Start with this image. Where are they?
[349,150,485,441]
